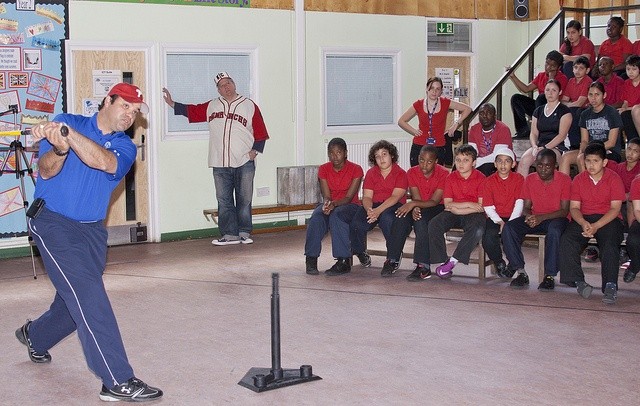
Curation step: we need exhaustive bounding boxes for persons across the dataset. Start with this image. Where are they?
[397,76,473,168]
[616,53,639,139]
[624,174,640,283]
[559,141,629,304]
[558,81,625,178]
[615,137,640,265]
[505,49,564,139]
[596,56,624,108]
[16,82,165,402]
[161,71,269,246]
[382,144,449,282]
[502,147,574,293]
[592,16,634,79]
[355,140,406,269]
[304,136,364,275]
[481,148,525,280]
[516,79,573,178]
[560,56,594,146]
[467,103,515,175]
[559,19,596,78]
[429,144,488,280]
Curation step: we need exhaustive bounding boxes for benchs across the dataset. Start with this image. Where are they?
[203,203,321,237]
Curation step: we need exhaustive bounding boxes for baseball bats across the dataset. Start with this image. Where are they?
[3,127,71,136]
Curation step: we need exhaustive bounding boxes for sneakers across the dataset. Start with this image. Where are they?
[306,256,319,274]
[602,282,617,303]
[584,247,598,262]
[325,258,351,275]
[502,262,516,278]
[407,263,431,281]
[241,236,253,243]
[497,267,506,277]
[538,275,555,291]
[357,251,371,267]
[212,238,240,245]
[436,259,455,276]
[100,376,163,401]
[576,281,592,298]
[381,260,395,276]
[15,318,51,363]
[510,273,529,289]
[440,263,452,279]
[623,267,636,282]
[395,251,403,270]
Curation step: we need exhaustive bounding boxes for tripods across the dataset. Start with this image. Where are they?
[0,103,38,280]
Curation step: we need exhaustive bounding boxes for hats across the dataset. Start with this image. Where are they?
[105,82,149,114]
[495,147,514,162]
[214,72,230,84]
